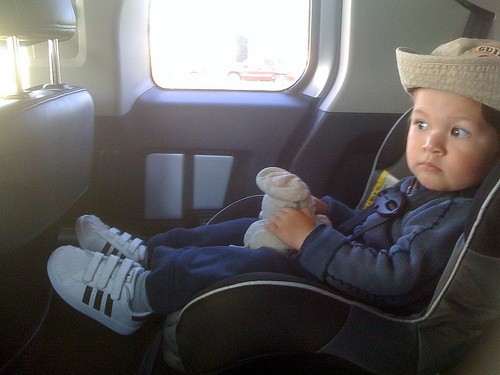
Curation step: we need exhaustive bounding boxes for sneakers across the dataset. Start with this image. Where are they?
[75,214,148,266]
[46,244,153,336]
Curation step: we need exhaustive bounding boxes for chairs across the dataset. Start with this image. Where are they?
[0,0,100,296]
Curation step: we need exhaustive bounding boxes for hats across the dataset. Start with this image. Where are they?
[395,36,500,111]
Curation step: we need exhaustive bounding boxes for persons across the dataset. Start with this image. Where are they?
[45,35,499,337]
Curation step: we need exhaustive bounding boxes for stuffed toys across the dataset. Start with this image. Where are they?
[241,165,333,259]
